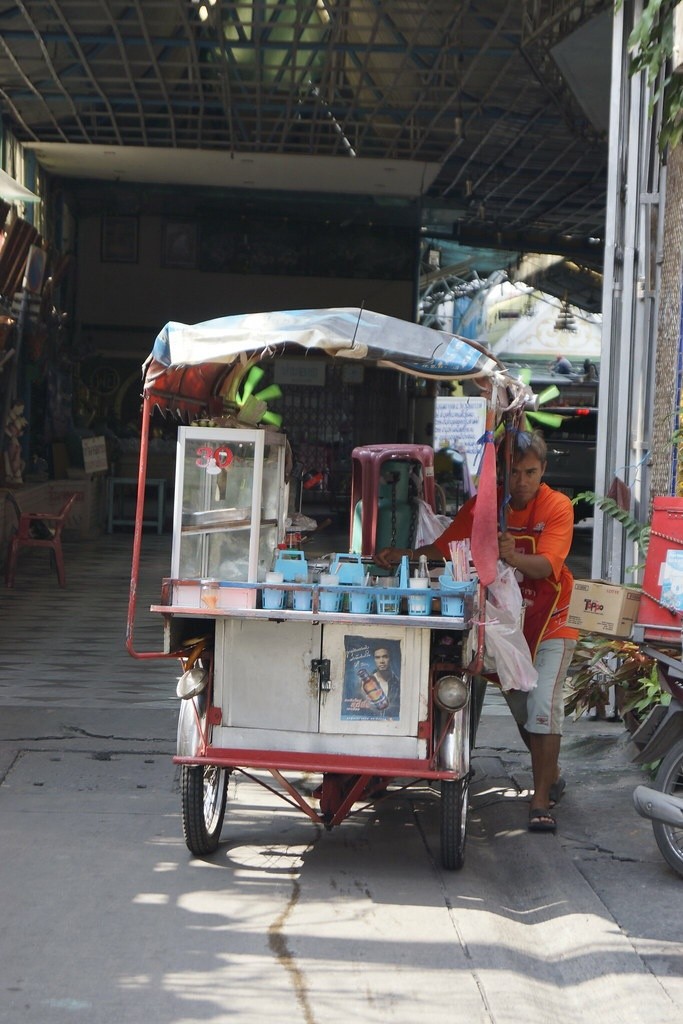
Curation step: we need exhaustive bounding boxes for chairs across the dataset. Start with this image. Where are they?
[6,489,80,588]
[434,453,460,517]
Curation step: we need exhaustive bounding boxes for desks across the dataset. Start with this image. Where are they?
[108,476,168,535]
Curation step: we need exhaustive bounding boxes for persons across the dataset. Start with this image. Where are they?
[373,431,574,831]
[360,646,399,705]
[5,400,25,484]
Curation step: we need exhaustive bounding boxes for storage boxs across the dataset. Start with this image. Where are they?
[564,579,642,636]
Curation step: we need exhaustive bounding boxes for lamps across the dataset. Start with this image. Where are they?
[558,358,599,379]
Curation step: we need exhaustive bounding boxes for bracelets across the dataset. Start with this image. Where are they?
[408,549,415,560]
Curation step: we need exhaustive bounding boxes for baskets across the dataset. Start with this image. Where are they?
[262,562,478,614]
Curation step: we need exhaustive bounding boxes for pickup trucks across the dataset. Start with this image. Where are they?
[533,405,597,503]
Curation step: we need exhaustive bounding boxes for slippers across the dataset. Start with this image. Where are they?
[528,808,556,829]
[549,778,565,808]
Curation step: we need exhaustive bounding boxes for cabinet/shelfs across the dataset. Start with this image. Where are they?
[171,426,293,610]
[212,619,431,760]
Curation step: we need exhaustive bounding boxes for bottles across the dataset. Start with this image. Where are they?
[200,580,221,609]
[278,544,291,559]
[328,553,336,574]
[270,548,280,572]
[76,379,90,416]
[417,555,431,588]
[408,577,428,612]
[285,533,297,560]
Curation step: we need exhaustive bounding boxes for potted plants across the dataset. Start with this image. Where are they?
[562,633,673,782]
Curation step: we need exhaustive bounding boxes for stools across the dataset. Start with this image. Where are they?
[350,444,435,557]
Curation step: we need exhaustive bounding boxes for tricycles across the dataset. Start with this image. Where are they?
[125,303,526,868]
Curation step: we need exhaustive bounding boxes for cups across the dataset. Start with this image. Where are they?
[318,574,340,611]
[264,572,283,608]
[377,577,399,614]
[351,574,372,613]
[294,573,313,610]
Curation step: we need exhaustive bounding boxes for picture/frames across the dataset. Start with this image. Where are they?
[101,214,140,264]
